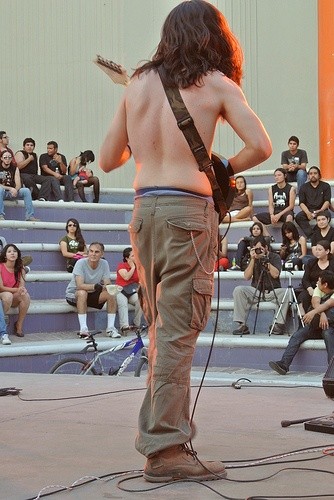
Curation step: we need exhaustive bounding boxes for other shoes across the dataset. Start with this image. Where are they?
[264,235,275,243]
[136,324,148,337]
[93,199,99,203]
[73,185,77,189]
[119,325,136,336]
[27,216,39,221]
[269,323,284,334]
[67,264,74,273]
[39,197,45,201]
[24,265,30,274]
[307,234,313,242]
[69,200,74,202]
[14,321,24,337]
[78,181,82,186]
[0,214,5,219]
[233,325,249,335]
[58,199,64,202]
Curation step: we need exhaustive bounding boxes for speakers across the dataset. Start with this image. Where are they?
[322,355,334,397]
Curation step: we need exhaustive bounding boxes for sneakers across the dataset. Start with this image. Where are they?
[268,360,287,375]
[106,326,121,338]
[79,327,89,340]
[0,333,11,345]
[143,441,227,482]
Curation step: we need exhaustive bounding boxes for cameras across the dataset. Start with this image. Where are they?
[255,248,263,254]
[284,262,293,268]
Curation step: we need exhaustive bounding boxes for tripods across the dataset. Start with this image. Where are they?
[240,264,285,337]
[269,273,305,337]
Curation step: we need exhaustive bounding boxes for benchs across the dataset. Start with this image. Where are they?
[0,169,334,372]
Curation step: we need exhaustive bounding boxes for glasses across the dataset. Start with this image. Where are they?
[1,156,12,160]
[68,224,77,228]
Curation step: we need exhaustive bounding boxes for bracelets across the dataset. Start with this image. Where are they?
[127,144,131,155]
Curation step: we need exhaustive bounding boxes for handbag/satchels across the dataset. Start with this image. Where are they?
[122,282,139,297]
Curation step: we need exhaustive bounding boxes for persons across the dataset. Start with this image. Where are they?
[99,0,272,481]
[252,167,296,241]
[0,244,30,344]
[295,166,331,241]
[232,237,290,335]
[116,247,142,326]
[281,136,308,196]
[215,235,230,270]
[297,240,334,328]
[0,150,39,220]
[269,271,334,375]
[222,176,253,222]
[0,131,100,203]
[65,242,121,339]
[59,218,87,273]
[231,211,334,271]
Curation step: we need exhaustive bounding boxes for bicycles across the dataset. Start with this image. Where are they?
[47,323,151,377]
